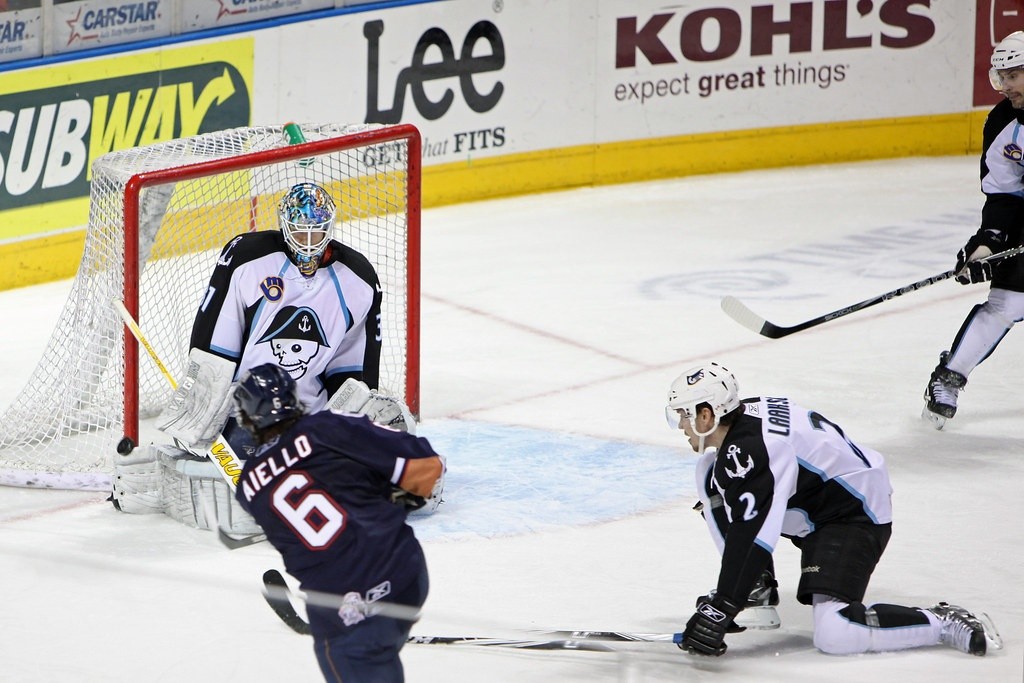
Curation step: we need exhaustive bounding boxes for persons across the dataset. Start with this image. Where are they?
[920,30,1024,431]
[665,363,1003,659]
[233,364,441,683]
[188,183,416,436]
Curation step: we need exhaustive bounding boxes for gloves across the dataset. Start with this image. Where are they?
[680,596,737,655]
[954,228,1007,286]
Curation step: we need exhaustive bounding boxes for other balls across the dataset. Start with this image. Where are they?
[116,437,135,456]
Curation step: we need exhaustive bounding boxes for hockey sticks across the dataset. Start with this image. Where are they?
[722,245,1024,338]
[217,524,268,550]
[261,567,684,654]
[108,296,245,501]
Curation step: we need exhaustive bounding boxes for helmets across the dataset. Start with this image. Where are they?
[231,364,302,441]
[988,31,1024,90]
[663,362,741,418]
[278,181,338,276]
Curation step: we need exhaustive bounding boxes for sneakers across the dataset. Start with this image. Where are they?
[695,569,780,628]
[920,350,966,430]
[927,601,1004,656]
